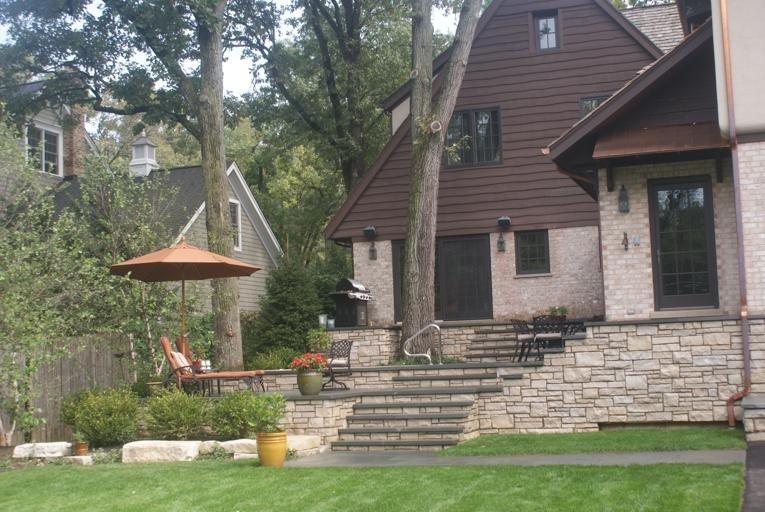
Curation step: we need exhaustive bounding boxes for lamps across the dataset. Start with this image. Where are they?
[369,242,377,260]
[498,216,511,225]
[497,233,505,251]
[363,226,376,235]
[618,184,629,212]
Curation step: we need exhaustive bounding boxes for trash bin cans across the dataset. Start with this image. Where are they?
[328,277,372,328]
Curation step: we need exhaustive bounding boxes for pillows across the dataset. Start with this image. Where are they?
[171,352,193,375]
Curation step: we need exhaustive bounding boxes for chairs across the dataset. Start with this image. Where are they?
[318,340,353,389]
[175,341,266,392]
[510,315,570,363]
[161,336,257,396]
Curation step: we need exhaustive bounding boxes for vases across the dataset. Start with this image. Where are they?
[298,369,323,395]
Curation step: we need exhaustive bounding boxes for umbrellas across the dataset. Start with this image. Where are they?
[108,239,262,357]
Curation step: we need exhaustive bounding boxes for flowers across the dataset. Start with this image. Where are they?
[288,352,328,371]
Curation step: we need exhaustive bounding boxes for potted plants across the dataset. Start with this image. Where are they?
[248,393,288,467]
[72,432,89,456]
